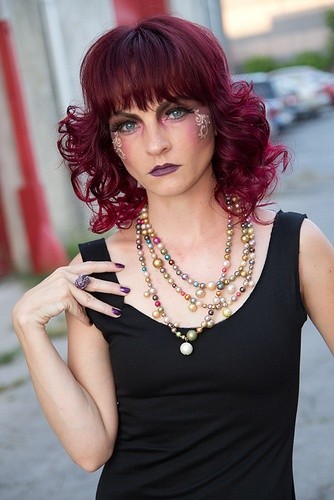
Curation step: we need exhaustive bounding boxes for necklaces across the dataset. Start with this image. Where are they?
[135,187,256,355]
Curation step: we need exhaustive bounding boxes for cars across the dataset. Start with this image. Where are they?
[234,64,333,139]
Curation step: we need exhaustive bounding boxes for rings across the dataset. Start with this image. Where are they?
[74,275,91,290]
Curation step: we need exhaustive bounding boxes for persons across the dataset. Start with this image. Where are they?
[8,11,333,499]
